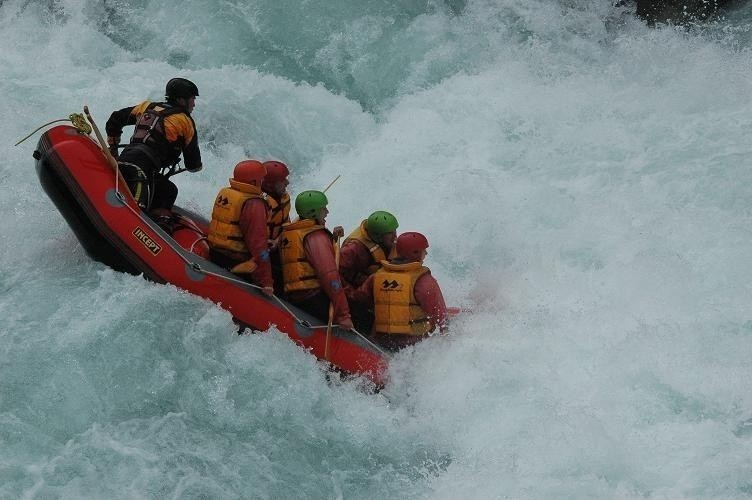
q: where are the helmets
[259,160,290,198]
[295,190,328,222]
[396,231,429,262]
[365,211,398,244]
[165,78,199,108]
[233,160,267,190]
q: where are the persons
[262,161,291,244]
[339,232,449,350]
[207,160,273,297]
[106,77,202,210]
[339,211,399,288]
[281,190,354,332]
